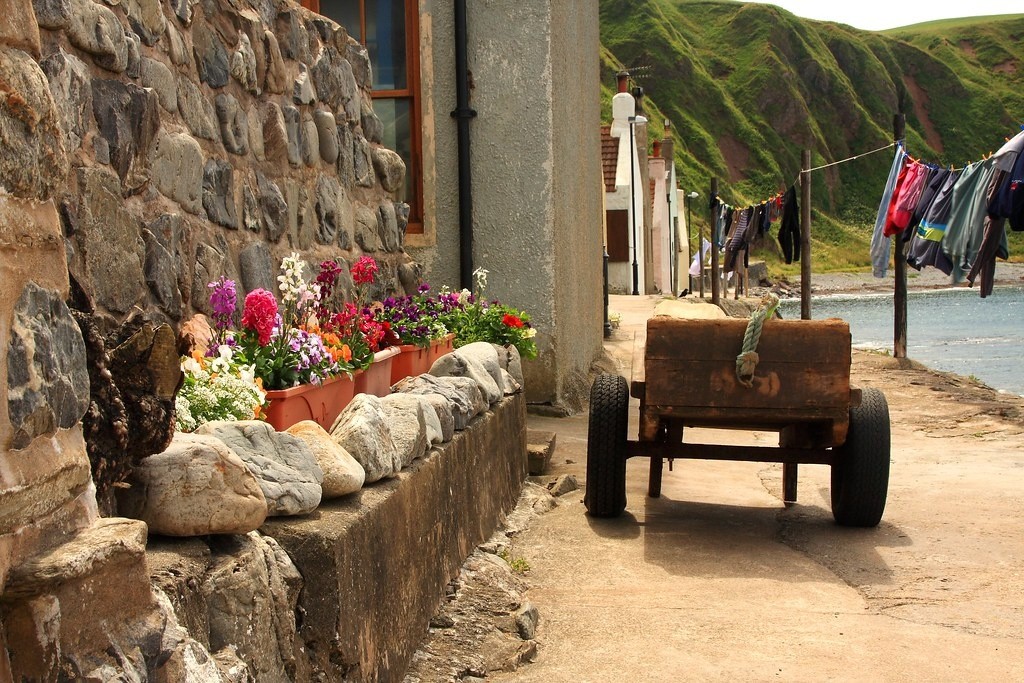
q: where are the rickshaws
[583,314,891,526]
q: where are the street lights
[688,192,699,294]
[628,116,647,296]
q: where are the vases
[391,334,456,385]
[353,346,401,398]
[262,368,364,432]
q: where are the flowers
[173,253,538,433]
